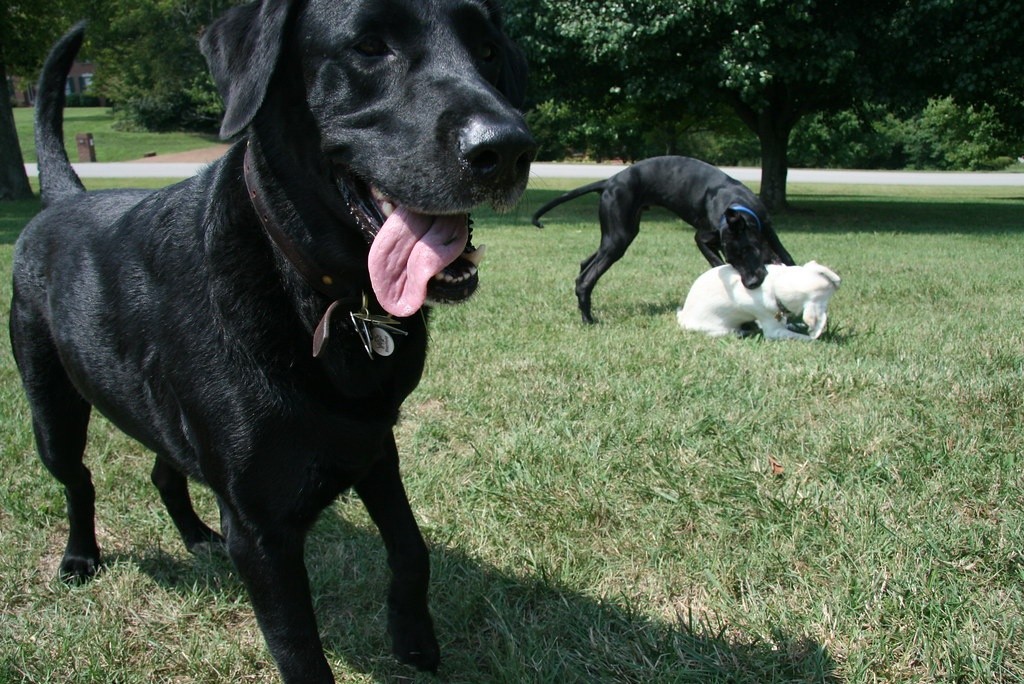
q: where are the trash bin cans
[75,132,96,162]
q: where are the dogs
[532,156,851,350]
[0,0,552,684]
